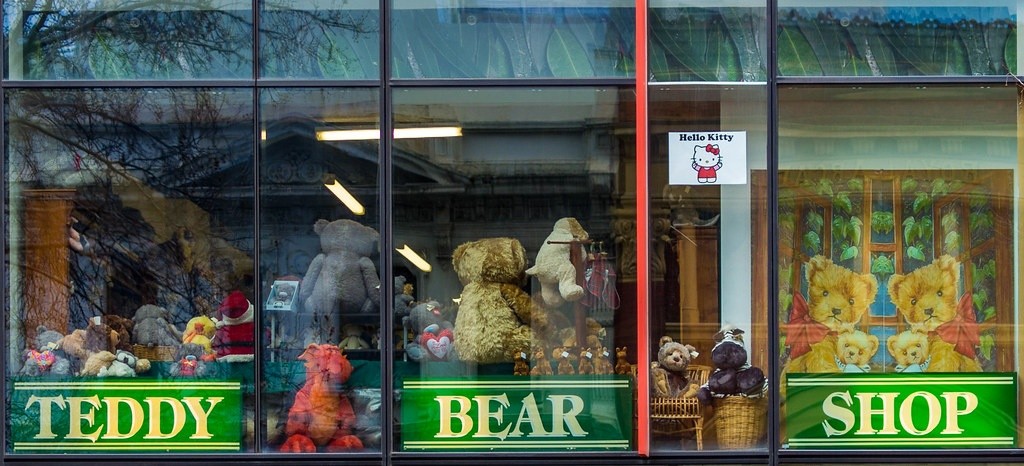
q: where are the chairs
[631,360,712,452]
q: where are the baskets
[133,345,179,361]
[712,396,768,450]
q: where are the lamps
[324,178,365,215]
[395,241,433,273]
[313,120,462,141]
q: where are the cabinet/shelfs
[21,189,109,347]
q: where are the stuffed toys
[395,217,632,375]
[652,326,765,400]
[22,289,254,377]
[282,343,363,453]
[262,219,380,349]
